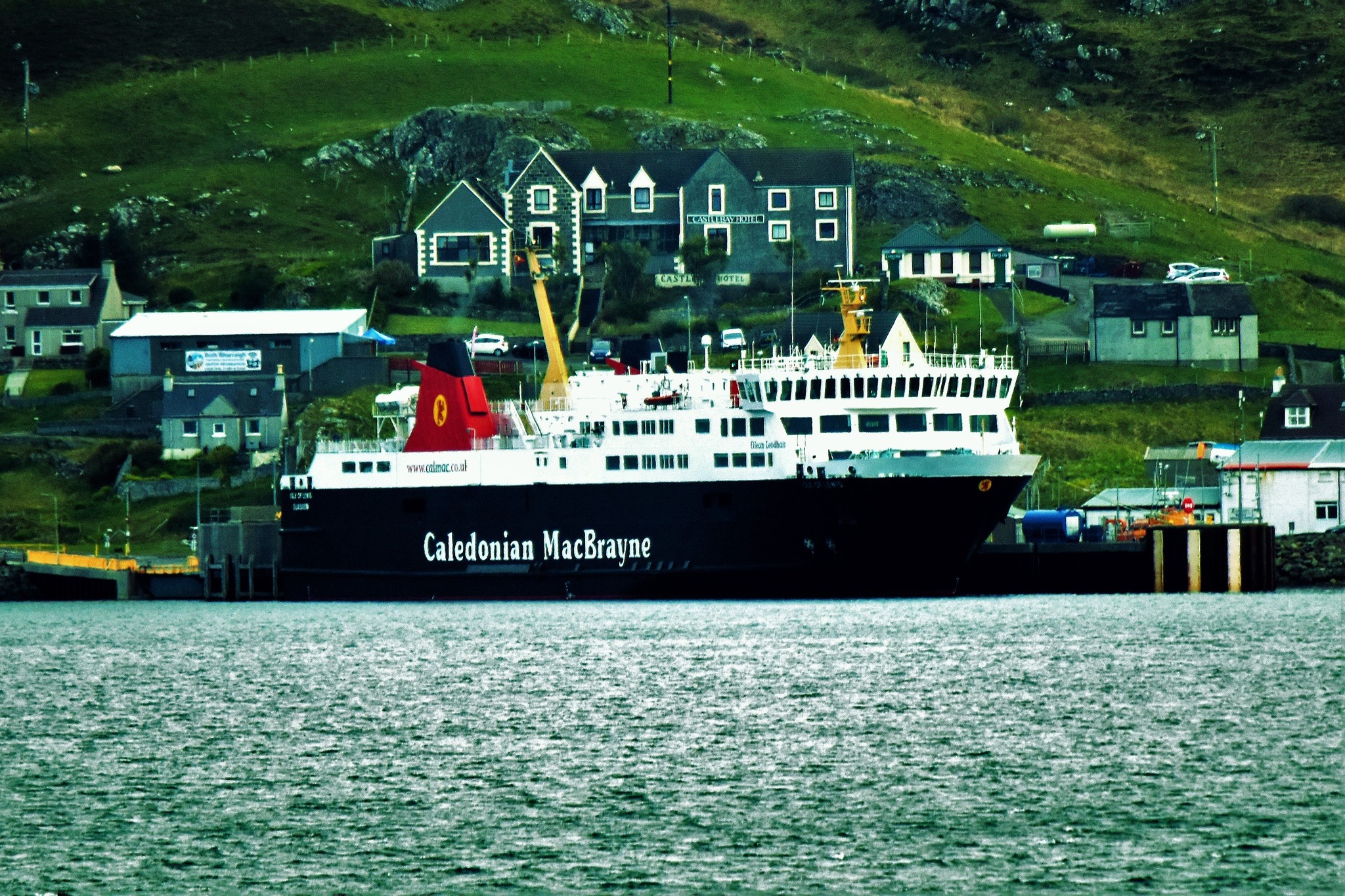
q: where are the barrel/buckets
[1021,506,1083,543]
[1089,525,1103,542]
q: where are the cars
[759,329,777,343]
[590,340,614,364]
[511,339,549,361]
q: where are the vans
[719,328,746,354]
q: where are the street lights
[1200,124,1224,219]
[684,296,691,361]
[39,493,59,565]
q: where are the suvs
[1174,267,1230,283]
[1165,263,1199,280]
[464,333,510,357]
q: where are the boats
[644,393,680,406]
[269,236,1045,602]
